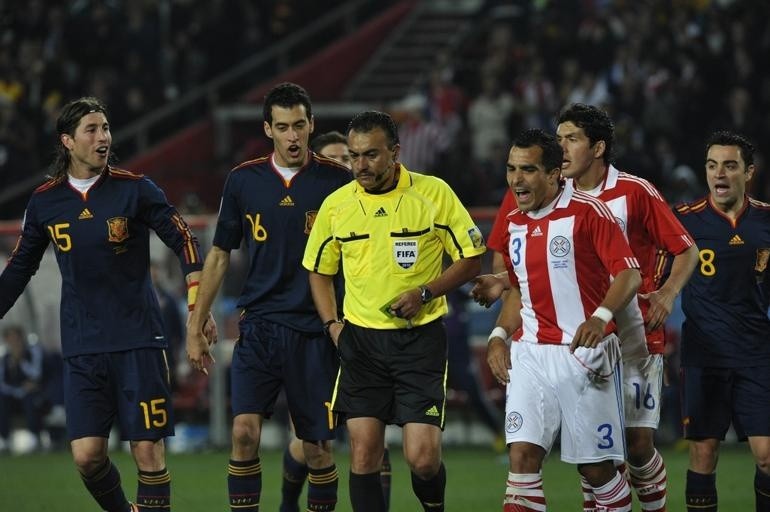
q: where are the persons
[0,324,50,455]
[279,131,391,511]
[188,82,353,512]
[468,106,700,511]
[657,133,770,512]
[0,98,218,511]
[0,1,149,187]
[302,111,485,511]
[146,259,188,397]
[485,131,644,512]
[396,0,770,209]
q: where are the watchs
[418,284,432,304]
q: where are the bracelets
[321,316,343,335]
